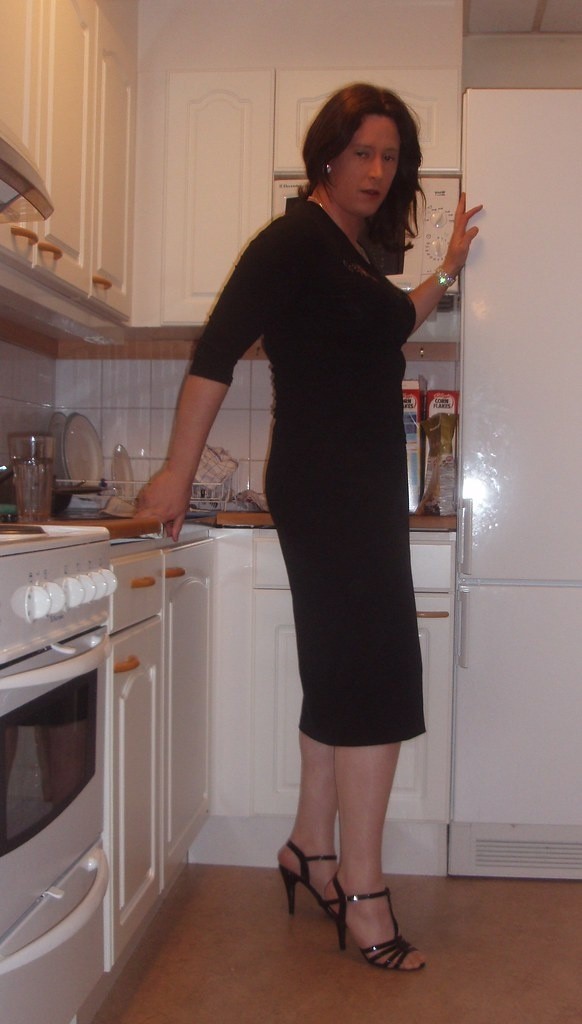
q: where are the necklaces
[308,196,324,210]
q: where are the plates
[111,444,134,495]
[47,409,66,476]
[63,413,104,483]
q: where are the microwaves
[276,178,460,294]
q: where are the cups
[9,435,52,521]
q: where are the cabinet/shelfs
[130,0,464,340]
[1,0,136,364]
[96,526,218,970]
[239,526,453,826]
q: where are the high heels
[324,875,425,972]
[277,840,343,922]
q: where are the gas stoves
[0,520,116,675]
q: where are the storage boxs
[404,374,462,516]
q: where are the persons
[133,83,483,971]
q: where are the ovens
[0,621,124,1024]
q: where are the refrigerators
[448,90,582,882]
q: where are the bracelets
[436,268,455,286]
